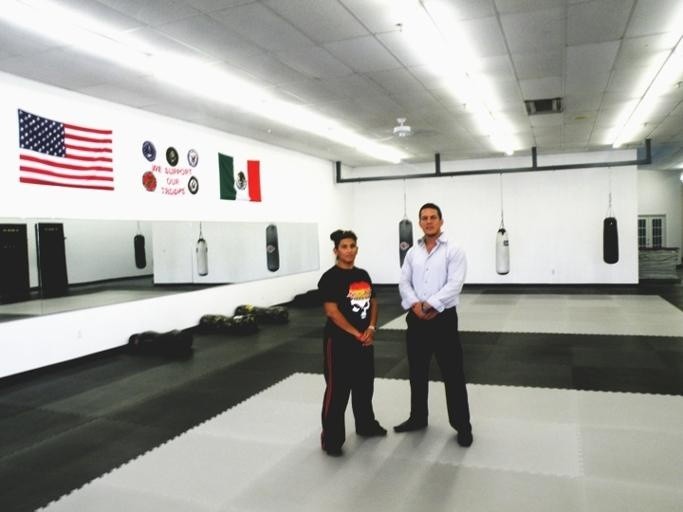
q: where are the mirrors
[0,216,321,325]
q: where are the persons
[317,230,387,457]
[392,203,473,447]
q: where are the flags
[17,109,114,190]
[217,151,261,202]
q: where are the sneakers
[326,446,343,455]
[367,425,386,436]
[394,418,428,432]
[457,425,472,446]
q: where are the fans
[368,118,443,144]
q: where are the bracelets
[355,332,362,342]
[367,325,377,332]
